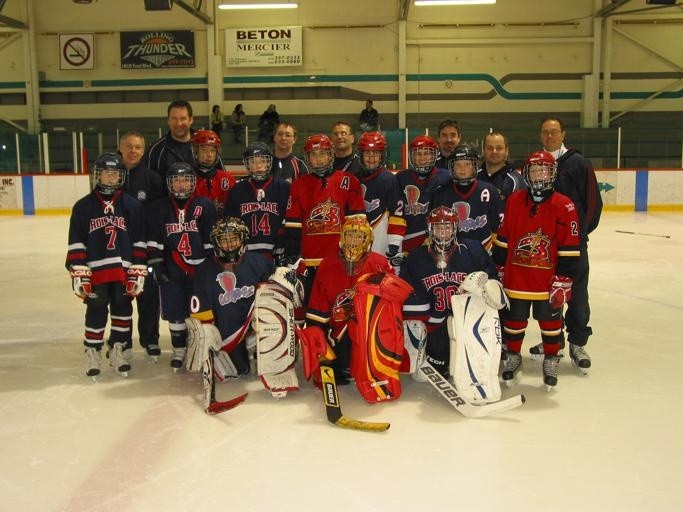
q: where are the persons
[145,101,227,180]
[210,103,280,144]
[224,141,292,260]
[189,129,238,219]
[498,116,602,384]
[105,131,165,356]
[146,161,218,367]
[357,97,380,126]
[352,115,529,274]
[187,216,278,379]
[265,120,363,180]
[396,206,501,382]
[303,216,398,389]
[66,150,146,376]
[283,132,368,310]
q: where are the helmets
[91,153,126,194]
[209,217,250,265]
[425,205,459,246]
[340,216,374,262]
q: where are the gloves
[548,274,575,309]
[146,257,170,290]
[69,265,98,303]
[122,264,148,298]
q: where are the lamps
[217,1,299,10]
[414,1,497,7]
